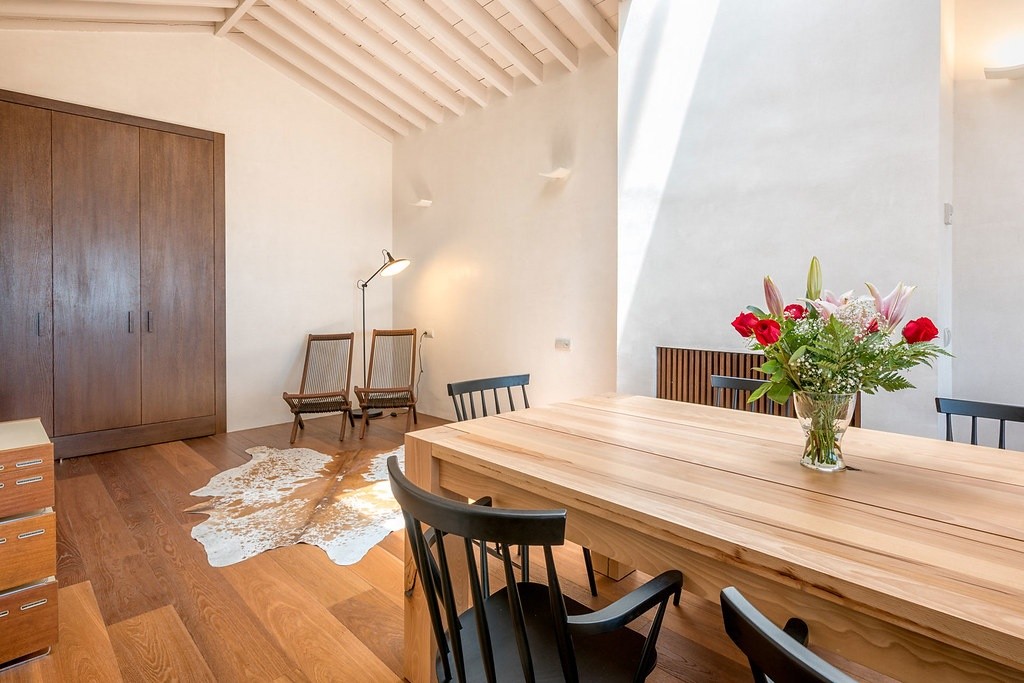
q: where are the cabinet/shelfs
[0,417,60,673]
[0,89,228,460]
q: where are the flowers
[731,256,955,466]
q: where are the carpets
[183,444,406,565]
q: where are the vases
[791,389,858,473]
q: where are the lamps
[350,249,410,418]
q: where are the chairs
[720,586,858,683]
[935,397,1024,451]
[447,376,598,602]
[387,455,684,683]
[282,333,355,443]
[353,328,417,440]
[672,375,790,607]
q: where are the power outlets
[424,330,433,338]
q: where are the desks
[402,392,1024,683]
